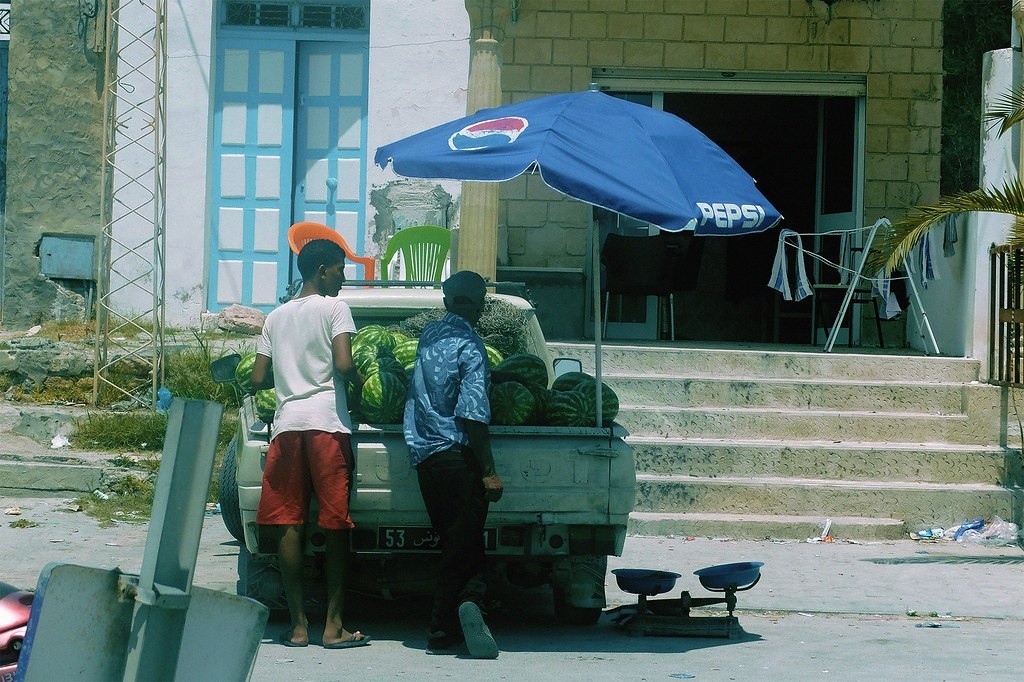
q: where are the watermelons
[234,324,621,425]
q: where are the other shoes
[458,602,500,660]
[426,630,470,655]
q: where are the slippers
[280,631,309,647]
[323,631,375,648]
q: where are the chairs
[380,225,451,288]
[288,222,375,289]
[810,248,884,350]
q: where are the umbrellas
[374,83,785,428]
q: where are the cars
[0,581,36,682]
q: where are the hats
[443,270,487,308]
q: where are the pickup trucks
[208,277,638,629]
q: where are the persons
[403,270,504,659]
[252,239,368,649]
